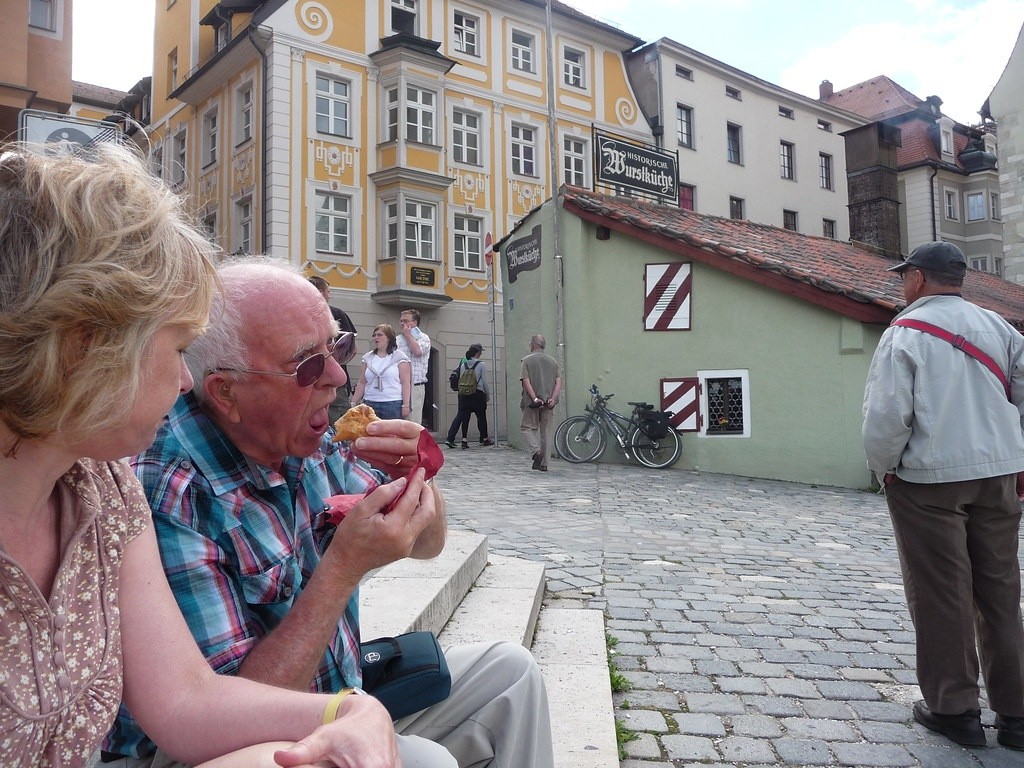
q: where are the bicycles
[555,384,683,468]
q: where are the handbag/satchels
[449,357,464,391]
[361,631,451,721]
[646,411,669,440]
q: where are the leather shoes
[993,713,1024,750]
[913,699,986,746]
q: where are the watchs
[322,687,368,724]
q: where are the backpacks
[458,361,482,396]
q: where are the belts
[414,383,424,386]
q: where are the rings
[394,455,403,466]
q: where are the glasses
[899,268,927,283]
[372,332,385,337]
[208,331,356,388]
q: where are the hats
[475,343,485,351]
[887,240,967,277]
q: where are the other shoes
[532,451,543,470]
[445,440,458,448]
[479,439,483,445]
[539,466,547,471]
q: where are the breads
[331,403,382,442]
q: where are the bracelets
[533,398,538,402]
[401,405,410,408]
[350,401,357,405]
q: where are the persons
[461,345,494,449]
[519,335,562,471]
[0,119,462,768]
[309,275,358,429]
[394,309,431,426]
[348,324,412,420]
[445,343,486,448]
[861,240,1024,749]
[100,253,557,768]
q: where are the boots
[483,439,495,446]
[461,441,470,449]
